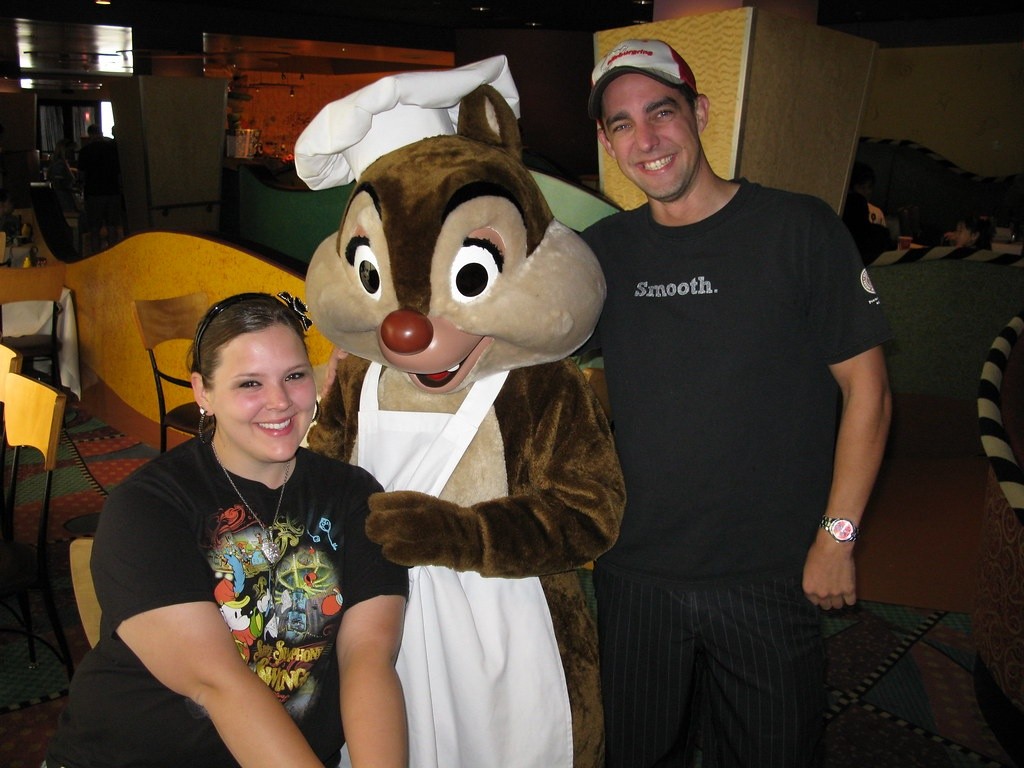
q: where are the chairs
[0,230,76,684]
[131,290,214,453]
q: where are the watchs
[818,515,861,544]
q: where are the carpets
[0,359,165,716]
[577,561,1023,768]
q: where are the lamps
[280,72,287,80]
[299,74,305,80]
[290,87,296,99]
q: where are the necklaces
[211,439,290,562]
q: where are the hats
[588,39,698,127]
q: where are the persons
[0,188,21,244]
[47,139,82,256]
[76,122,121,252]
[325,39,893,768]
[38,293,411,768]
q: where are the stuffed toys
[294,54,630,768]
